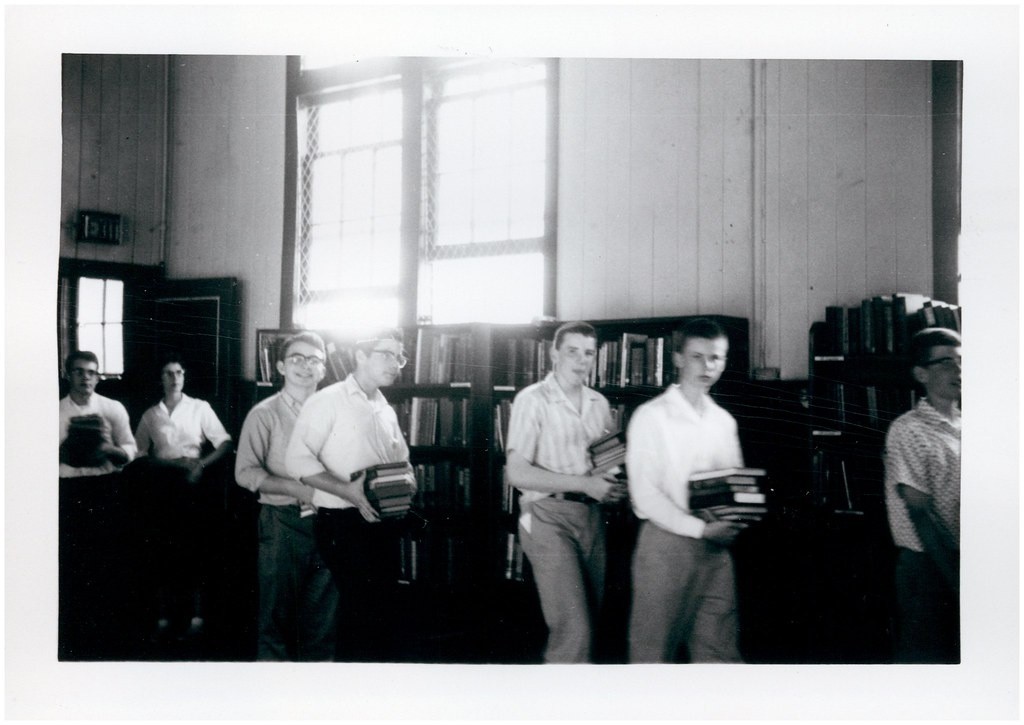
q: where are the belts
[548,491,599,507]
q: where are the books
[823,294,959,428]
[256,317,768,585]
[67,414,116,449]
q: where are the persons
[625,319,747,663]
[133,361,232,634]
[237,331,326,662]
[286,325,418,663]
[505,321,629,664]
[883,326,960,664]
[59,351,143,479]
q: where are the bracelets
[199,459,205,468]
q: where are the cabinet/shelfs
[253,313,750,583]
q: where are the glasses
[70,368,101,380]
[282,352,326,369]
[369,348,407,368]
[923,356,961,369]
[162,369,185,380]
